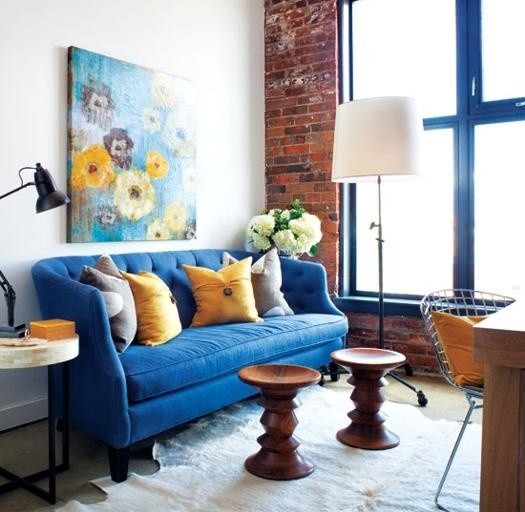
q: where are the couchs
[28,247,348,483]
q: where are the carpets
[52,384,482,512]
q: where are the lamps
[0,163,74,339]
[330,94,430,407]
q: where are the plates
[0,338,47,347]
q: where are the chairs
[417,286,518,512]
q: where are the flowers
[241,200,324,261]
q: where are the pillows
[221,246,295,317]
[120,268,184,347]
[179,256,264,330]
[434,306,495,390]
[75,251,139,356]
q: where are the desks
[1,332,83,507]
[472,291,525,511]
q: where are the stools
[332,345,409,452]
[234,361,321,482]
